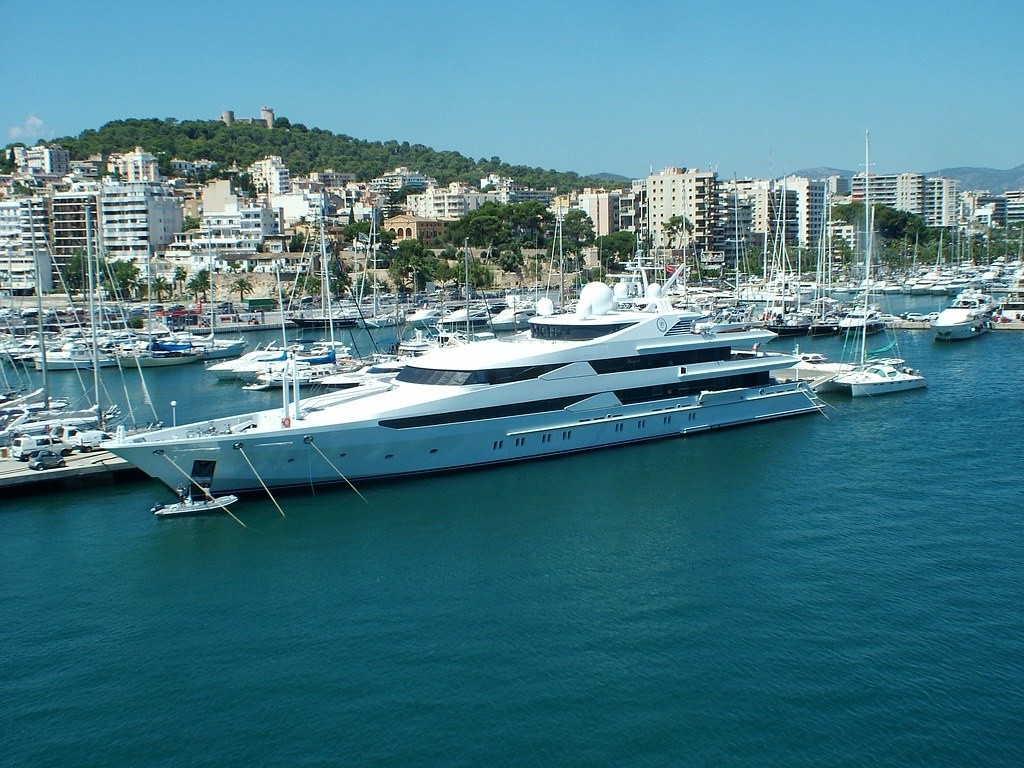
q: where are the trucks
[242,297,278,313]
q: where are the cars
[68,308,89,317]
[21,307,44,317]
[47,309,67,316]
[300,296,320,310]
[144,304,164,313]
[429,293,445,302]
[330,294,346,304]
[282,298,294,310]
[217,301,233,308]
[361,293,375,304]
[413,291,428,302]
[95,307,111,315]
[126,306,146,315]
[169,304,185,312]
[484,294,495,299]
[378,292,397,306]
[398,291,411,304]
[188,303,205,313]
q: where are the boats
[0,128,1024,520]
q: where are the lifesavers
[283,418,291,427]
